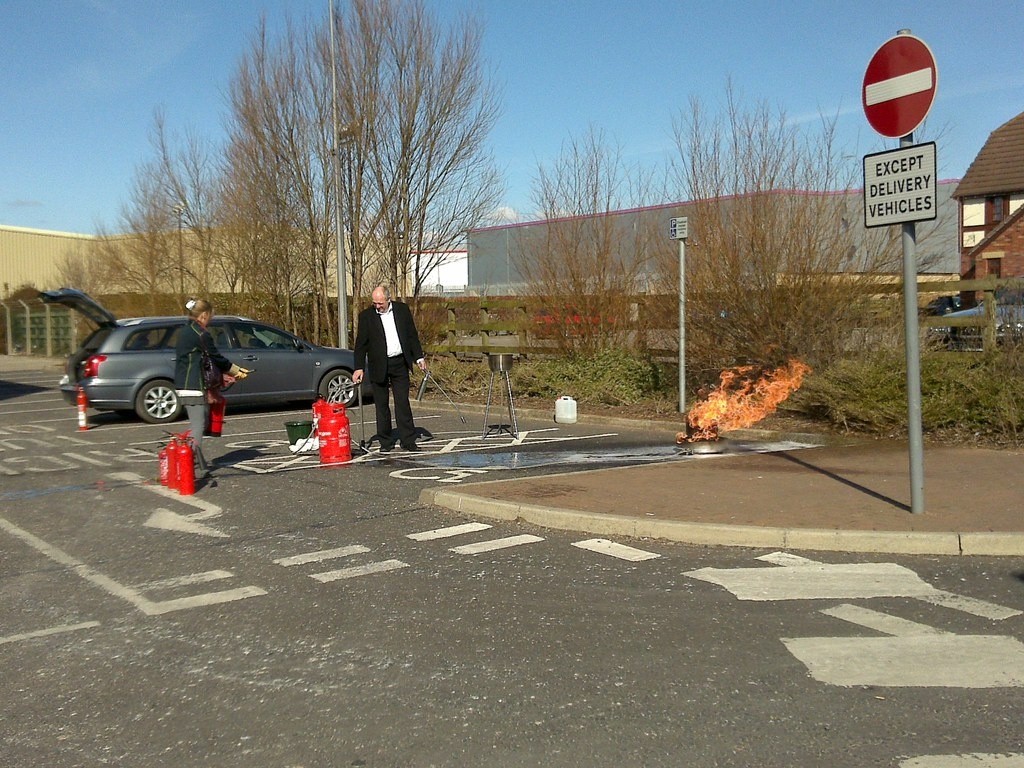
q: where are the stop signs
[858,33,940,141]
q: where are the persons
[352,286,427,451]
[174,300,249,479]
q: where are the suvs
[37,287,375,423]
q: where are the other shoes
[401,444,421,451]
[380,444,394,452]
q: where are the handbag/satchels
[199,331,223,390]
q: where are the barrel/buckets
[554,395,578,424]
[283,420,315,446]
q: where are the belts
[387,354,404,359]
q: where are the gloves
[226,363,249,380]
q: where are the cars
[923,285,1023,352]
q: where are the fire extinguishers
[156,428,196,496]
[76,385,89,431]
[203,368,257,437]
[415,369,467,425]
[313,392,353,464]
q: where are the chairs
[216,332,228,350]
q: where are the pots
[481,351,514,372]
